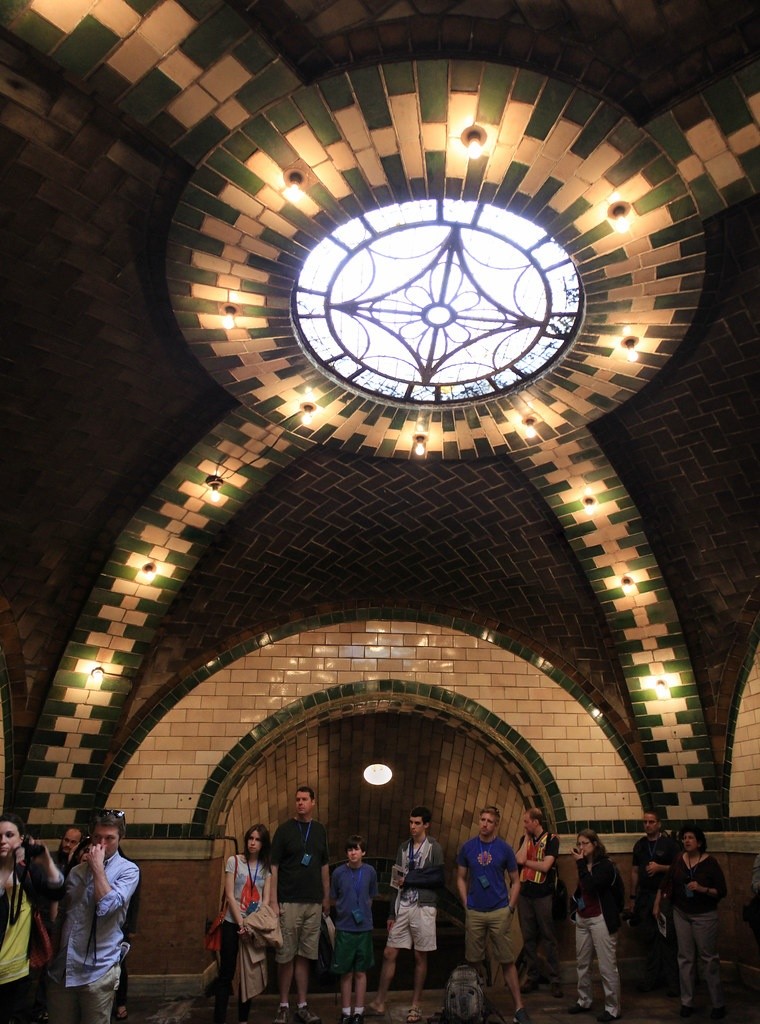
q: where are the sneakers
[513,1008,531,1024]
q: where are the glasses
[97,809,125,826]
[81,834,90,842]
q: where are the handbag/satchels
[205,911,226,952]
[17,863,52,968]
[548,833,568,921]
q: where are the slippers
[117,1006,127,1020]
[353,1003,385,1016]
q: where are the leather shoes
[569,1003,591,1014]
[680,1004,694,1017]
[667,983,680,997]
[340,1013,352,1024]
[637,979,661,991]
[352,1011,365,1024]
[520,978,539,994]
[597,1011,620,1021]
[711,1005,726,1018]
[551,982,564,998]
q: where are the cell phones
[573,848,578,854]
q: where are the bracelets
[509,906,515,913]
[706,888,710,893]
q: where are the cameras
[21,835,45,865]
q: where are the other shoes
[294,1005,322,1024]
[274,1005,290,1024]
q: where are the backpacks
[442,964,490,1024]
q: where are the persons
[456,806,532,1024]
[630,810,680,960]
[567,828,624,1023]
[652,827,730,1024]
[751,854,760,894]
[269,787,330,1024]
[514,808,565,999]
[363,806,446,1023]
[212,824,272,1024]
[331,835,379,1024]
[0,808,143,1024]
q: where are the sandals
[406,1007,423,1023]
